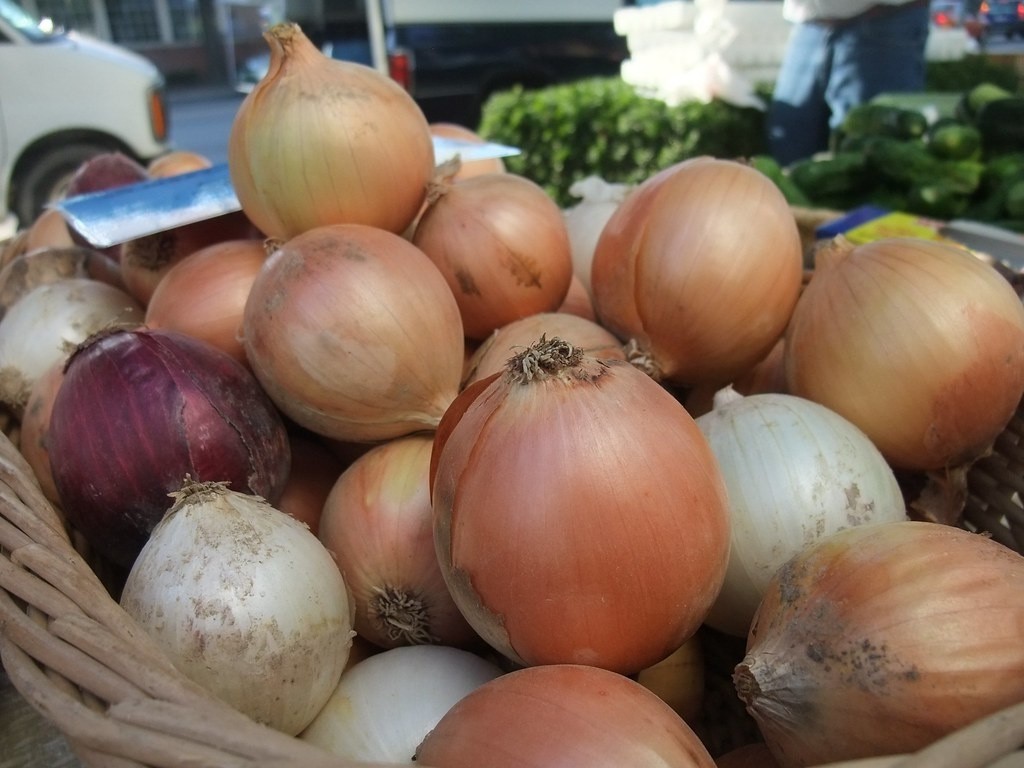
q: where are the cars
[1,0,170,225]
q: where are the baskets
[1,182,1024,768]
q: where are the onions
[0,20,1024,768]
